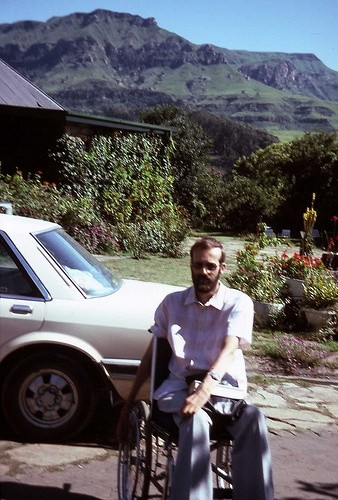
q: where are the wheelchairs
[116,335,234,500]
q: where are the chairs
[277,230,290,238]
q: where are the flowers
[282,252,324,279]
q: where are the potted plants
[251,276,284,327]
[299,277,338,327]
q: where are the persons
[115,235,276,500]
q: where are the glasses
[191,261,221,271]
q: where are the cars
[0,212,189,443]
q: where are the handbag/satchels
[188,379,248,427]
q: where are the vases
[284,277,304,299]
